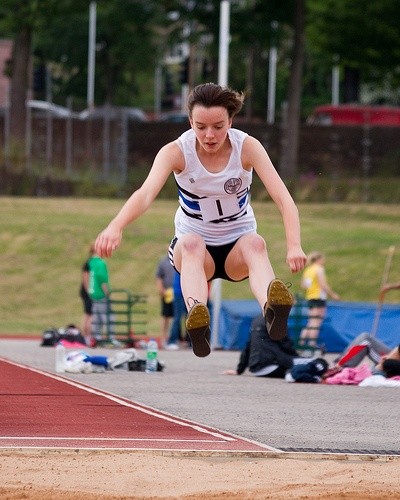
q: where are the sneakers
[186,302,212,358]
[264,278,295,340]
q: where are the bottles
[54,341,68,373]
[144,337,159,374]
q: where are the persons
[94,84,307,357]
[221,315,400,380]
[301,251,339,348]
[80,249,188,349]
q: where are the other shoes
[110,337,121,347]
[160,337,188,351]
[85,337,97,347]
